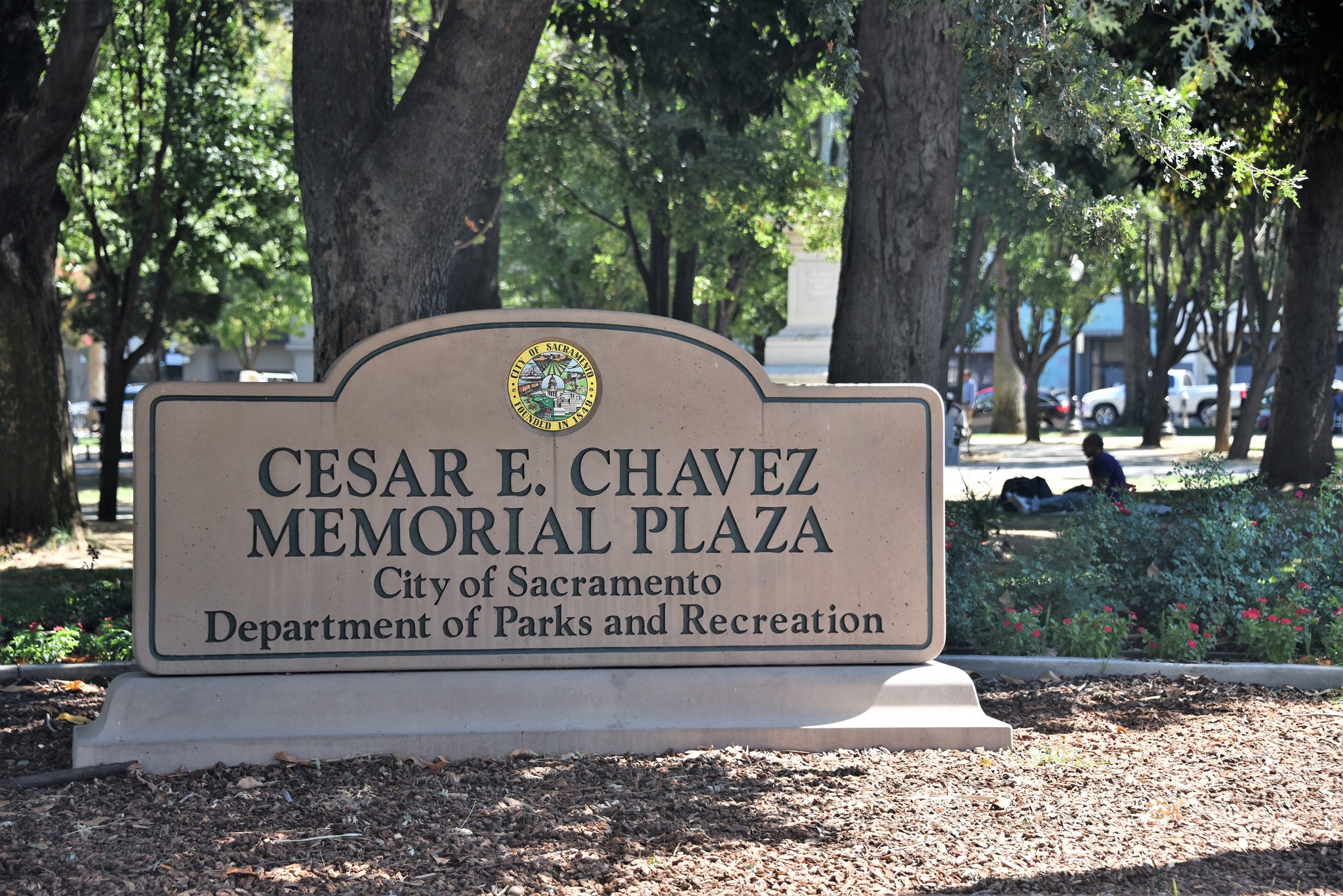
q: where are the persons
[1005,433,1126,516]
[960,369,976,439]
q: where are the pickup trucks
[1081,369,1246,429]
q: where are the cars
[946,384,1076,434]
[90,381,144,458]
[1204,379,1343,433]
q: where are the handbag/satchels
[1003,476,1053,501]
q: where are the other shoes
[1010,493,1030,515]
